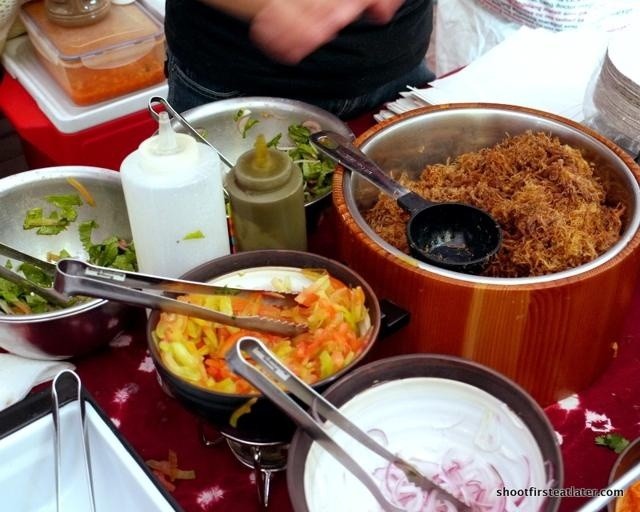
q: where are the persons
[163,0,436,124]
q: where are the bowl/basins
[144,253,381,427]
[341,106,640,286]
[154,97,358,212]
[287,352,564,511]
[0,164,127,362]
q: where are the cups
[43,0,112,27]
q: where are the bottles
[117,113,229,304]
[229,132,308,251]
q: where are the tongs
[46,257,311,342]
[0,241,69,307]
[48,370,97,511]
[227,334,471,512]
